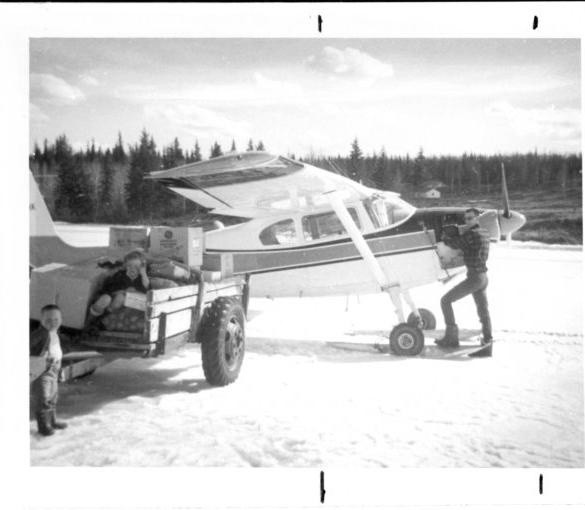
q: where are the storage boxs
[150,227,203,267]
[109,228,147,263]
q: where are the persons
[430,207,494,359]
[31,303,68,437]
[68,249,151,352]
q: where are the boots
[468,338,492,356]
[85,312,98,332]
[435,325,459,347]
[34,409,67,436]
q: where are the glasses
[462,216,476,220]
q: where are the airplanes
[30,150,527,358]
